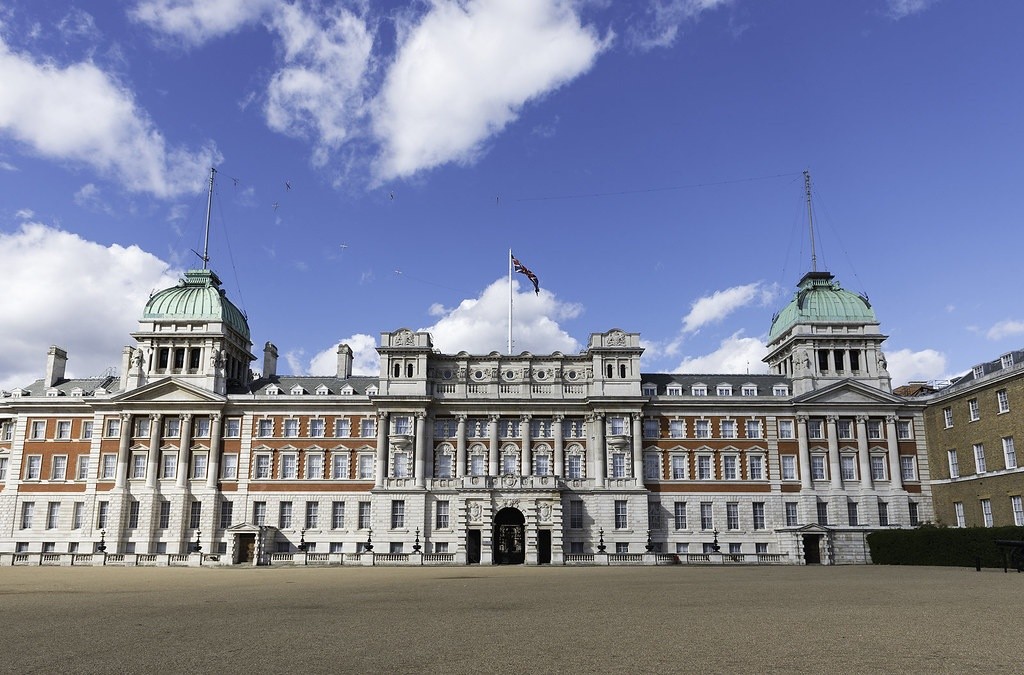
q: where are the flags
[511,256,540,296]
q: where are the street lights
[298,531,306,551]
[597,527,606,552]
[194,531,202,552]
[412,527,421,552]
[712,527,720,552]
[645,531,654,552]
[98,530,106,552]
[364,530,373,551]
